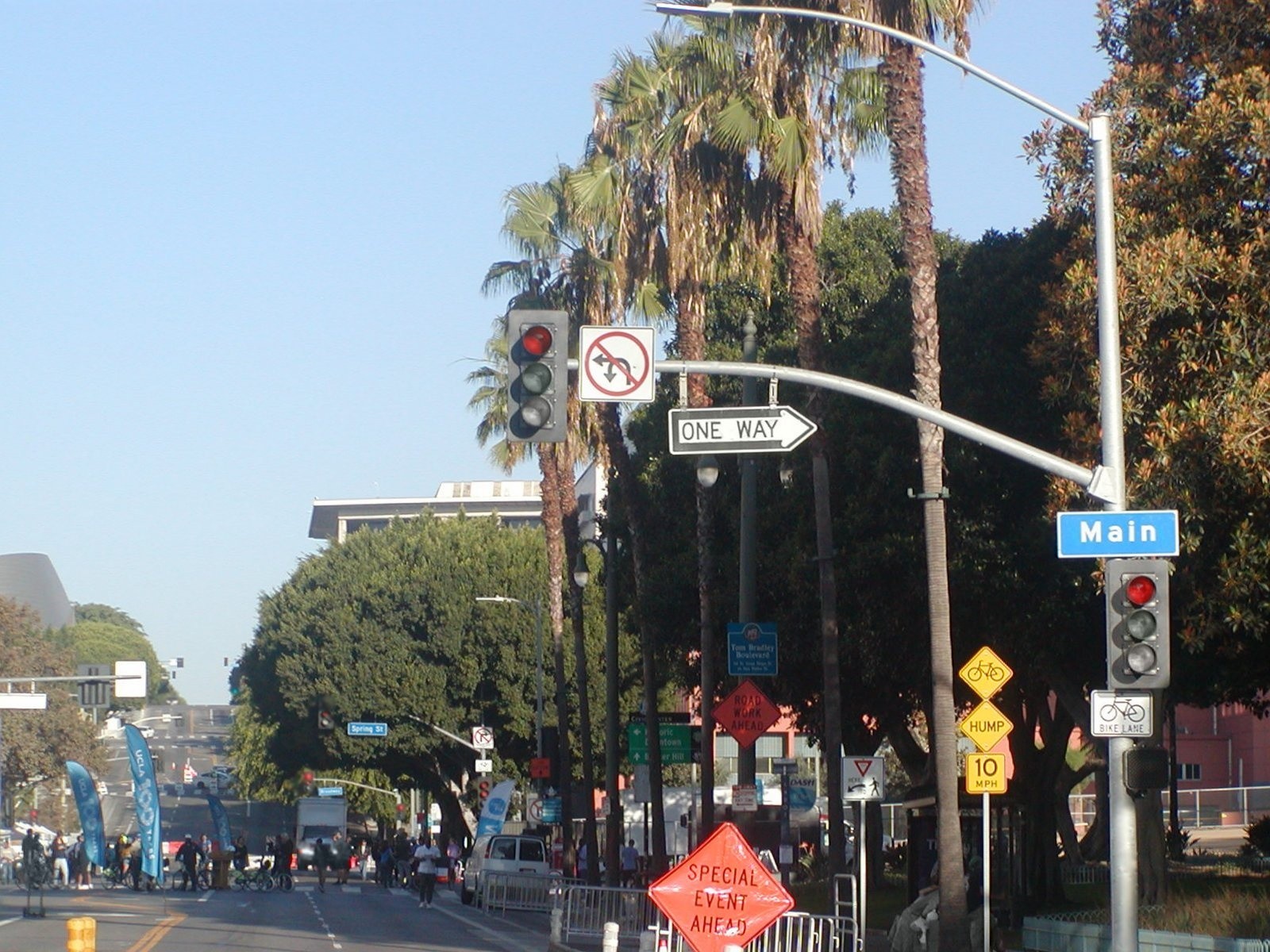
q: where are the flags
[65,760,107,867]
[206,794,232,850]
[125,722,164,886]
[476,780,516,835]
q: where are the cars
[193,763,241,790]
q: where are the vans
[461,835,548,905]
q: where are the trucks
[296,797,349,871]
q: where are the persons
[331,828,462,891]
[576,838,588,899]
[621,839,639,888]
[887,837,981,952]
[415,835,441,909]
[312,837,331,893]
[0,828,294,891]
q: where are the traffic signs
[629,724,692,749]
[841,755,884,804]
[667,406,818,456]
[629,713,692,723]
[629,746,693,764]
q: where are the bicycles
[228,850,295,892]
[12,852,68,891]
[101,855,213,892]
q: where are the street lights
[654,0,1142,952]
[474,595,545,836]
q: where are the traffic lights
[231,684,239,695]
[475,774,495,811]
[398,803,407,820]
[508,307,569,444]
[1105,559,1171,691]
[317,706,333,728]
[303,768,315,793]
[31,808,38,826]
[1123,743,1172,793]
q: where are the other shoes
[426,903,431,909]
[333,880,348,886]
[54,884,93,890]
[419,902,424,908]
